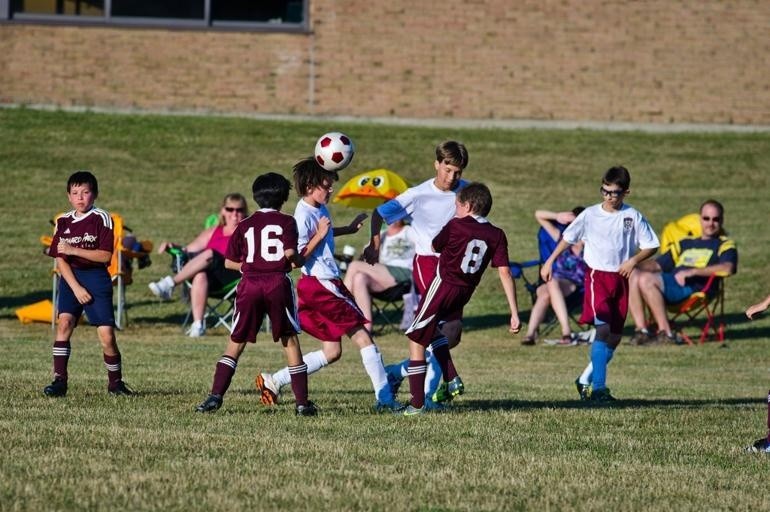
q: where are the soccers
[315,132,355,171]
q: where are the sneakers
[520,334,534,344]
[188,320,203,338]
[108,381,132,399]
[255,373,279,405]
[295,402,317,417]
[591,388,616,401]
[630,331,684,345]
[148,275,176,297]
[557,333,577,346]
[197,394,223,414]
[432,376,463,402]
[572,377,592,401]
[745,436,770,453]
[44,381,67,396]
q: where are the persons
[44,171,134,398]
[522,206,587,347]
[539,167,662,406]
[628,200,737,344]
[254,155,404,413]
[194,174,317,418]
[389,182,522,417]
[342,219,414,335]
[149,193,247,339]
[363,140,471,415]
[745,294,770,454]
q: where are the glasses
[701,215,721,222]
[223,206,246,212]
[599,186,625,197]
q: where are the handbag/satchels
[399,272,424,330]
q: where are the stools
[368,277,414,335]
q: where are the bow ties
[377,361,445,415]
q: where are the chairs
[649,212,735,342]
[39,210,153,331]
[509,223,591,340]
[166,212,252,335]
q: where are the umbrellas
[334,171,410,208]
